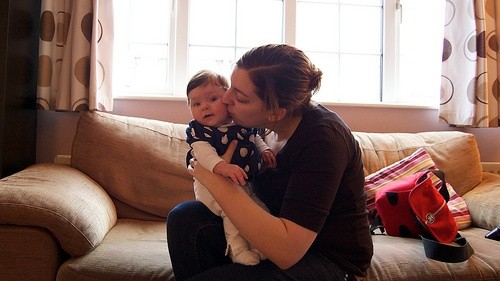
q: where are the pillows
[364,147,472,234]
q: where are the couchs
[0,112,500,281]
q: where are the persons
[167,43,374,281]
[185,70,278,266]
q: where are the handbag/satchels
[372,170,459,245]
